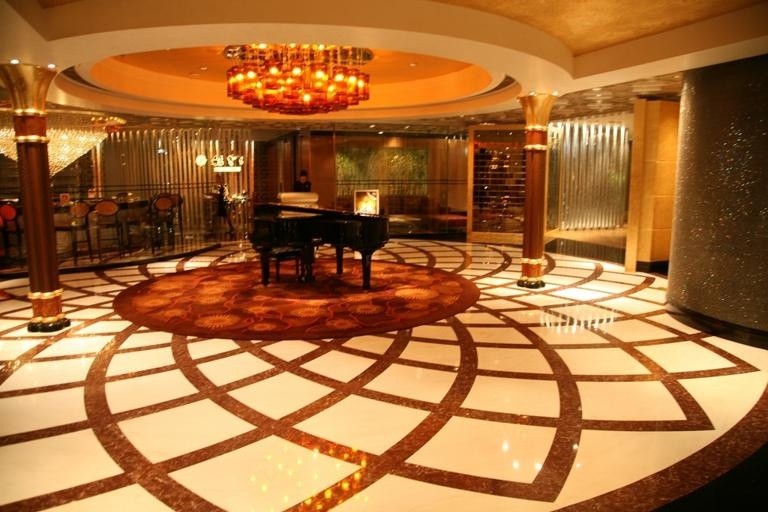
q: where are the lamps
[222,44,374,115]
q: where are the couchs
[337,195,467,238]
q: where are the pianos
[248,200,389,291]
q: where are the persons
[294,170,312,192]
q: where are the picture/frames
[354,189,379,215]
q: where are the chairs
[0,192,184,269]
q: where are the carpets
[111,256,481,341]
